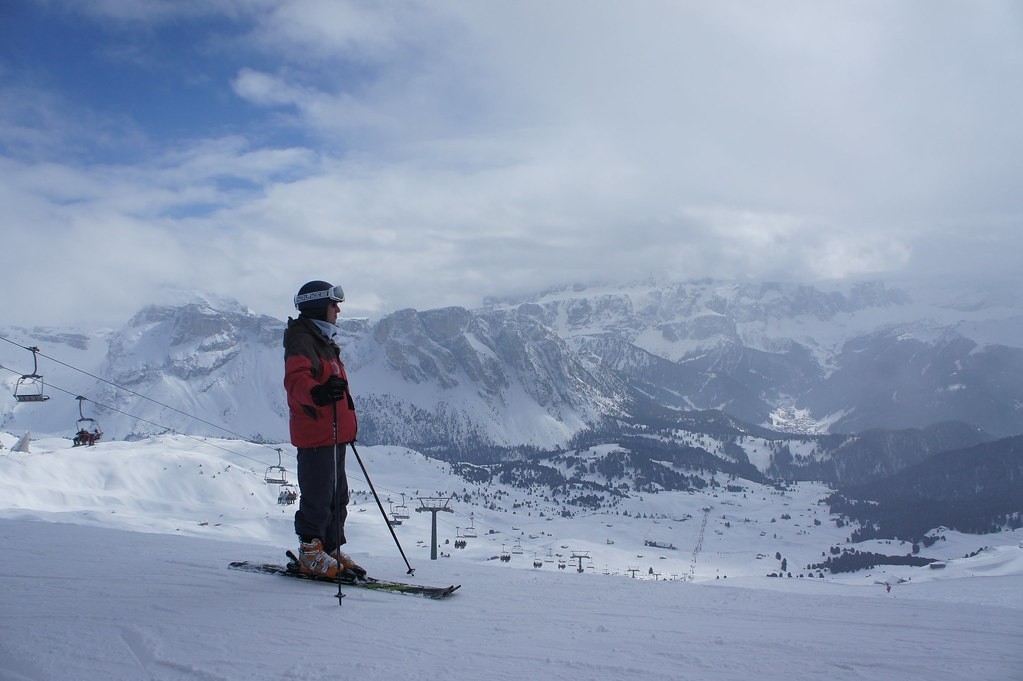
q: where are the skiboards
[227,561,461,598]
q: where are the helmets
[298,281,334,321]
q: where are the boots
[298,539,367,584]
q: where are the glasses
[295,285,345,305]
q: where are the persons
[278,490,297,506]
[454,539,466,549]
[558,563,566,569]
[73,428,101,445]
[283,280,366,581]
[500,555,511,562]
[534,561,542,568]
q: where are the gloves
[312,375,348,406]
